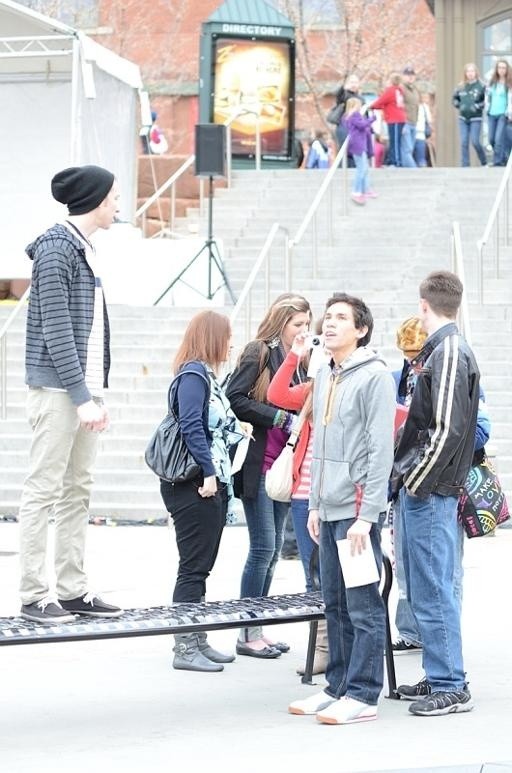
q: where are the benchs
[0,553,401,700]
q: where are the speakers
[194,122,227,179]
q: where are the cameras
[303,335,325,350]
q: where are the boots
[172,632,224,672]
[297,619,329,675]
[196,632,235,663]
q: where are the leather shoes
[268,641,290,652]
[236,638,281,658]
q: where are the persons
[452,63,481,167]
[289,294,396,724]
[300,66,435,206]
[483,60,511,165]
[160,312,253,671]
[387,271,479,719]
[146,112,168,154]
[19,165,124,624]
[226,293,314,660]
[265,320,336,677]
[387,315,490,658]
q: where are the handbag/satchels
[265,390,314,503]
[145,360,213,483]
[221,340,272,476]
[457,456,510,538]
[327,103,345,125]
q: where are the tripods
[151,181,238,310]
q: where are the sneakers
[350,190,378,203]
[315,695,377,724]
[57,591,125,618]
[397,672,467,701]
[20,596,76,623]
[384,637,423,656]
[409,681,474,716]
[288,689,339,715]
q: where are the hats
[403,68,415,75]
[396,317,429,351]
[51,165,114,216]
